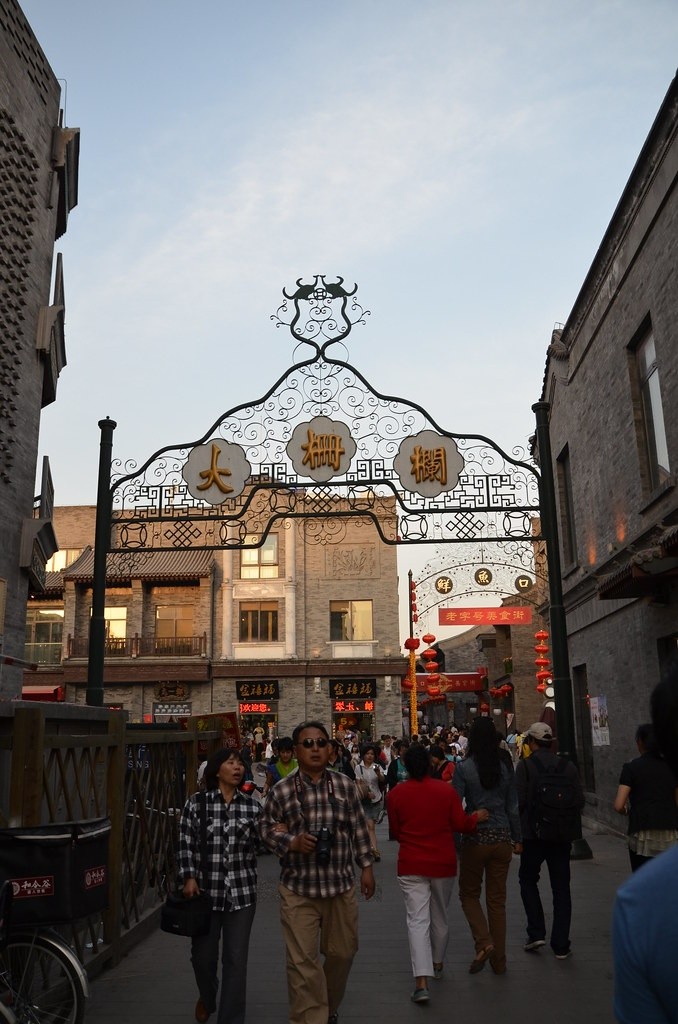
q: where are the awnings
[22,685,61,694]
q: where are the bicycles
[0,816,112,1024]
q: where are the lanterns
[422,634,436,643]
[489,684,512,698]
[422,648,437,658]
[402,678,414,687]
[425,661,438,672]
[412,582,418,622]
[534,629,551,692]
[427,674,440,683]
[418,686,446,708]
[405,638,420,648]
[481,704,489,711]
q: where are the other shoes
[432,966,444,978]
[328,1012,338,1024]
[489,954,506,975]
[371,847,381,862]
[469,943,494,974]
[411,988,430,1002]
[195,982,219,1023]
[375,811,386,824]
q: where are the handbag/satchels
[353,764,369,799]
[159,888,214,937]
[432,760,452,781]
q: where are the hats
[524,721,552,741]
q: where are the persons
[384,744,490,1002]
[516,721,579,960]
[241,693,533,857]
[259,721,376,1024]
[452,716,523,975]
[615,723,678,873]
[175,749,289,1024]
[610,668,678,1024]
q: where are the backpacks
[527,752,584,844]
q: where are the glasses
[297,737,329,748]
[473,715,494,722]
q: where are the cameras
[308,827,335,869]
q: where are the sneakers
[523,935,546,950]
[555,948,572,959]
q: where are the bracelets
[625,806,628,816]
